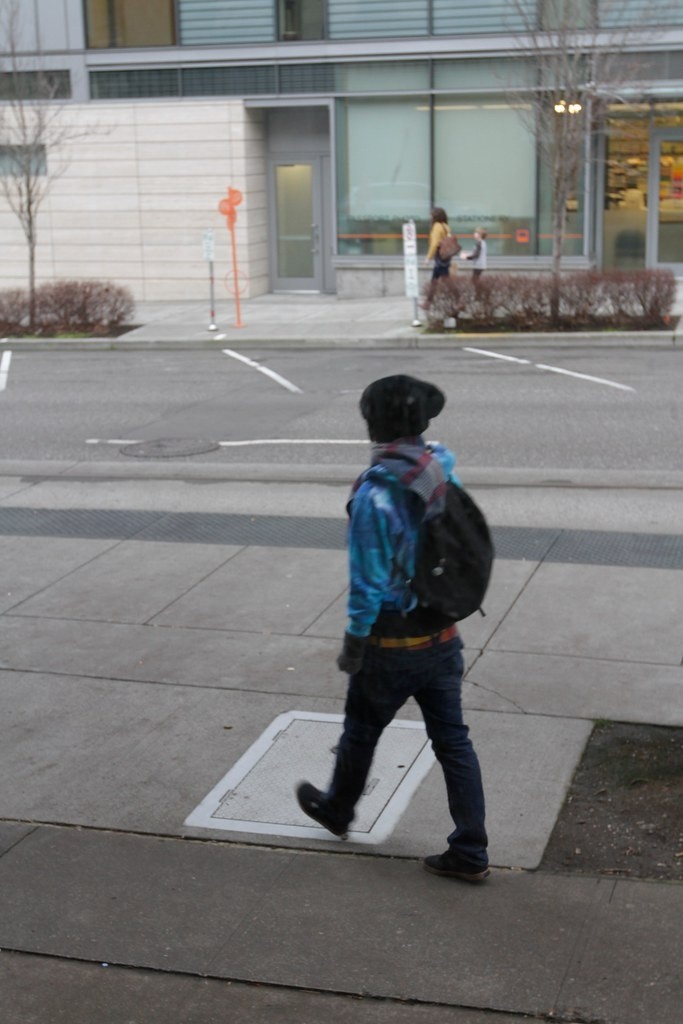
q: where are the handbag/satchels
[436,219,461,260]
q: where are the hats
[360,375,444,441]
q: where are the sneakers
[297,781,348,836]
[423,855,491,883]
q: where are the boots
[418,278,437,310]
[441,277,461,305]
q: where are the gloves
[337,631,369,674]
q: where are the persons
[293,372,491,884]
[459,227,488,301]
[416,207,465,311]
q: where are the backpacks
[396,480,495,638]
